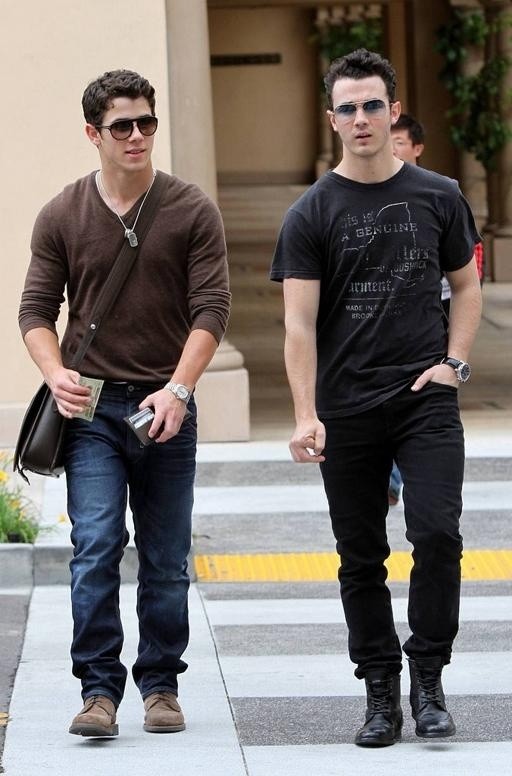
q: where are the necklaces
[96,166,159,251]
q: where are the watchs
[440,356,472,383]
[165,380,192,403]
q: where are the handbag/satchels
[12,380,69,486]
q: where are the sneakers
[69,695,119,737]
[142,692,185,733]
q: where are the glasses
[333,99,395,127]
[96,115,160,141]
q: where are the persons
[19,71,232,738]
[269,50,485,747]
[383,115,484,508]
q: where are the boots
[355,668,403,747]
[406,653,456,739]
